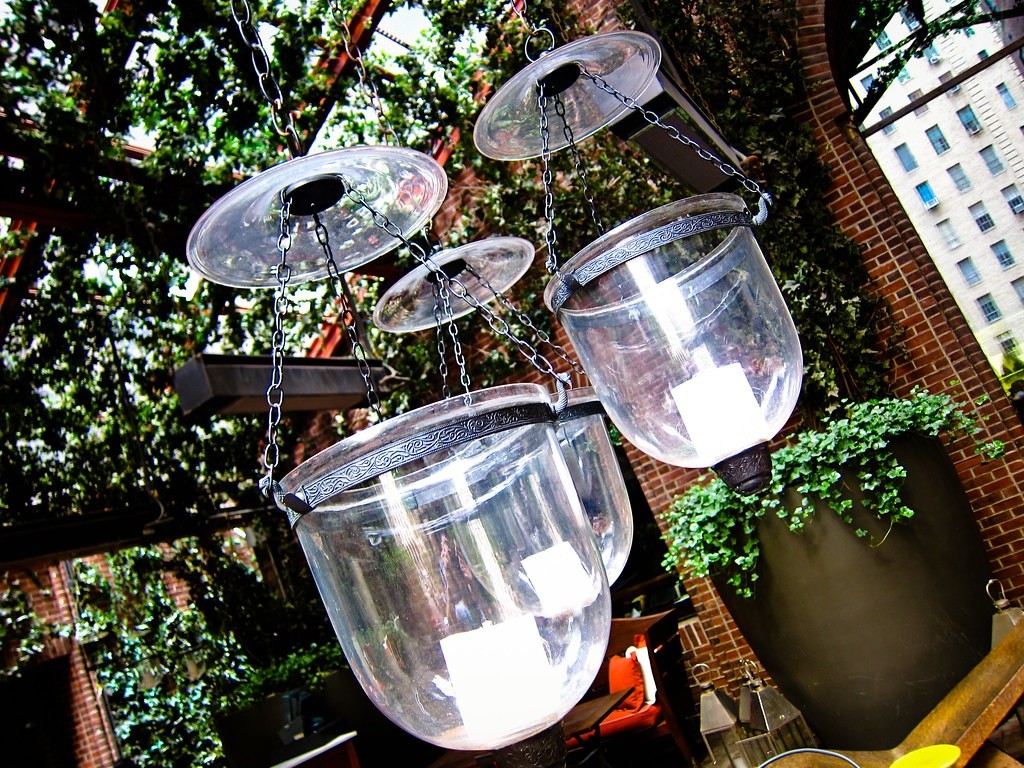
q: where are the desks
[427,687,635,768]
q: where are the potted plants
[661,389,1008,749]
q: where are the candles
[440,613,552,735]
[522,542,594,613]
[672,364,767,457]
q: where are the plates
[888,744,962,768]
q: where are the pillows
[609,646,658,711]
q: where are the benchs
[562,609,697,768]
